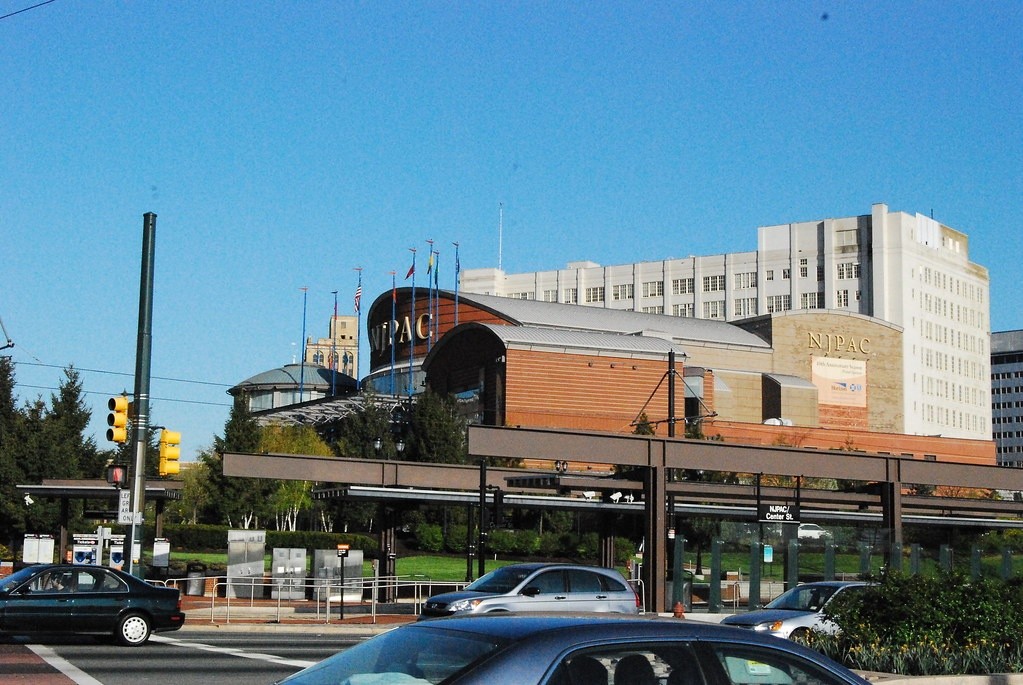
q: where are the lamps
[555,460,568,471]
[373,434,406,461]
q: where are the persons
[29,571,69,593]
[613,654,655,685]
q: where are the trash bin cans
[186,562,207,596]
[664,570,692,613]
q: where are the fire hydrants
[673,603,685,620]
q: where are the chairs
[568,653,660,685]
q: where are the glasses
[51,576,58,580]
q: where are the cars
[272,614,873,685]
[777,523,834,537]
[719,580,882,646]
[0,564,187,645]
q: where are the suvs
[416,562,641,617]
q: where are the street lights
[374,439,405,459]
[555,460,569,476]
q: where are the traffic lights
[107,396,128,443]
[158,428,181,475]
[107,467,129,485]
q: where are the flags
[353,280,361,313]
